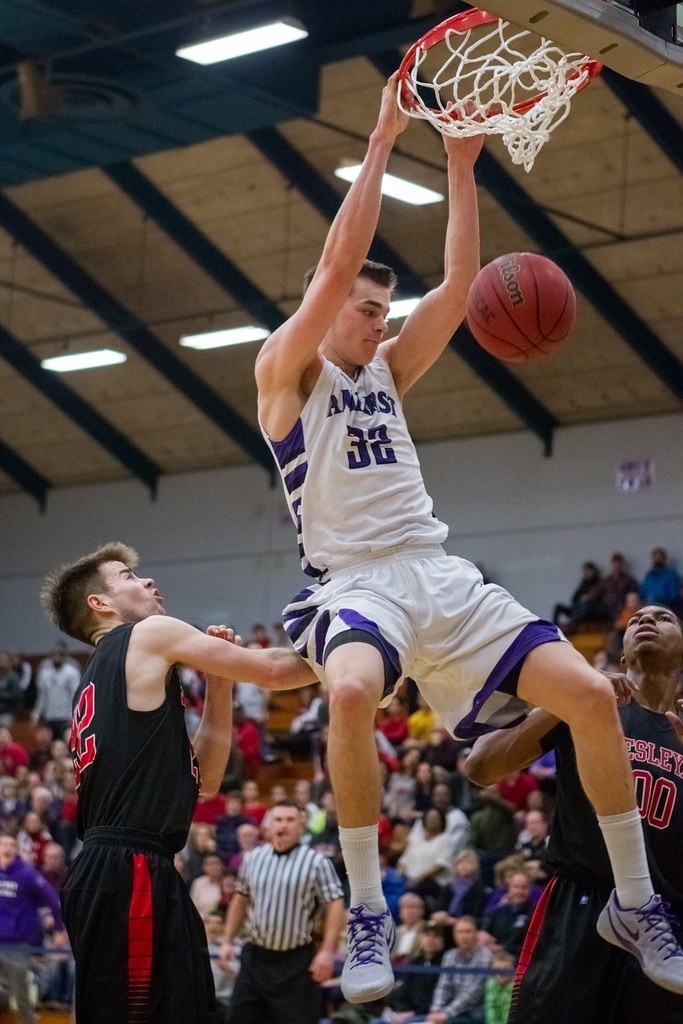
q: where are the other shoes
[36,1001,69,1011]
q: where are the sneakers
[341,903,398,1004]
[597,888,683,995]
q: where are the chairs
[8,687,324,844]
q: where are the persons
[465,604,683,1023]
[40,542,320,1024]
[170,623,561,1024]
[254,71,683,1005]
[472,546,683,718]
[0,639,81,1024]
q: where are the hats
[422,920,443,934]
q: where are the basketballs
[465,250,578,364]
